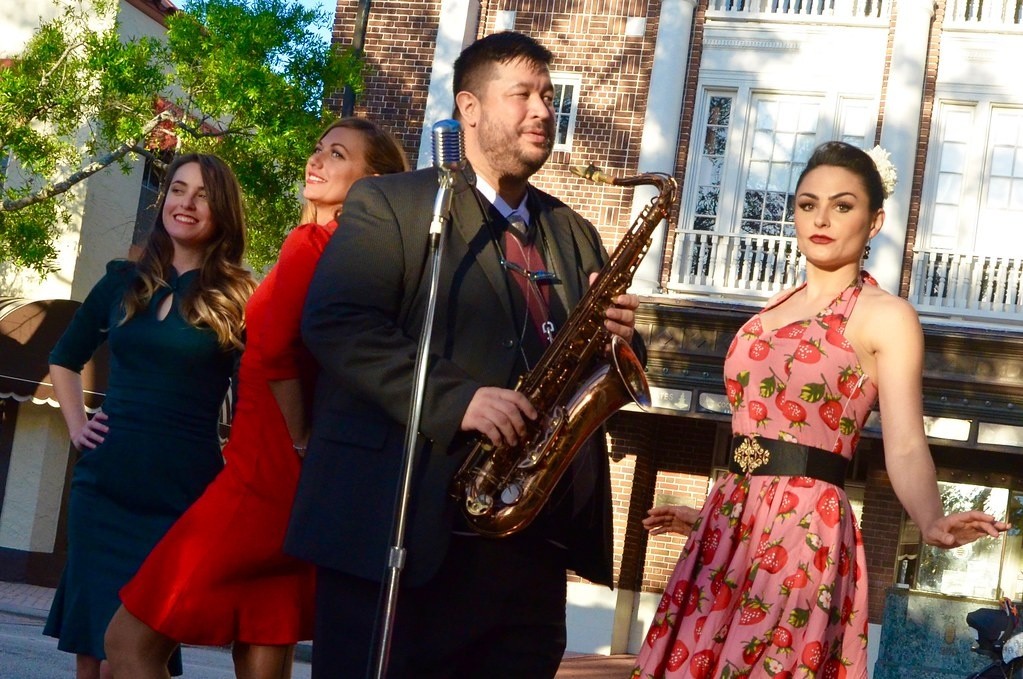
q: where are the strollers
[964,597,1023,679]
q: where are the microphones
[429,119,476,252]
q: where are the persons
[967,597,1023,648]
[282,32,647,679]
[105,117,407,679]
[44,153,259,679]
[629,141,1012,679]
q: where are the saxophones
[442,161,684,540]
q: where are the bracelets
[293,445,307,450]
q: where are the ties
[509,210,527,233]
